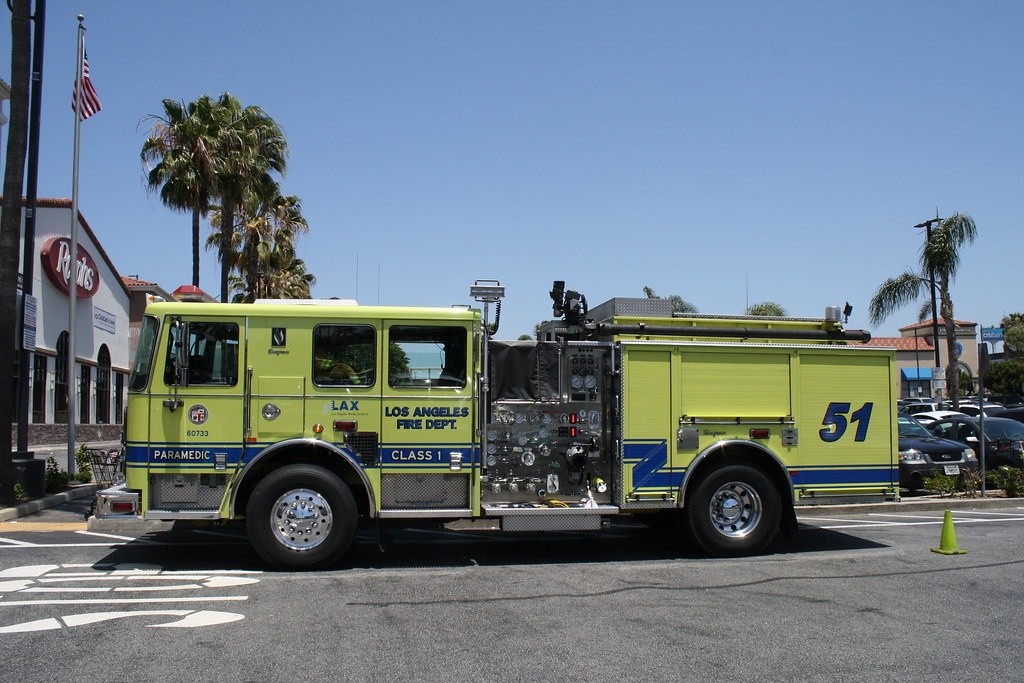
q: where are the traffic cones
[930,509,968,554]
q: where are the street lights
[914,218,946,412]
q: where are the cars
[897,395,1024,443]
[896,410,981,498]
[923,416,1024,489]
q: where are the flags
[70,25,101,121]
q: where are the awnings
[902,367,932,379]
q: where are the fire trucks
[92,279,903,572]
